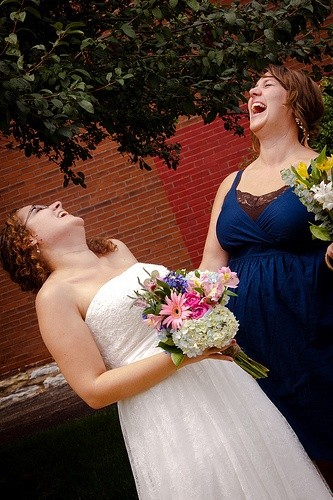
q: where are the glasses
[23,205,49,226]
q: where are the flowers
[279,144,332,270]
[124,266,270,380]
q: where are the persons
[196,68,333,486]
[0,200,333,500]
